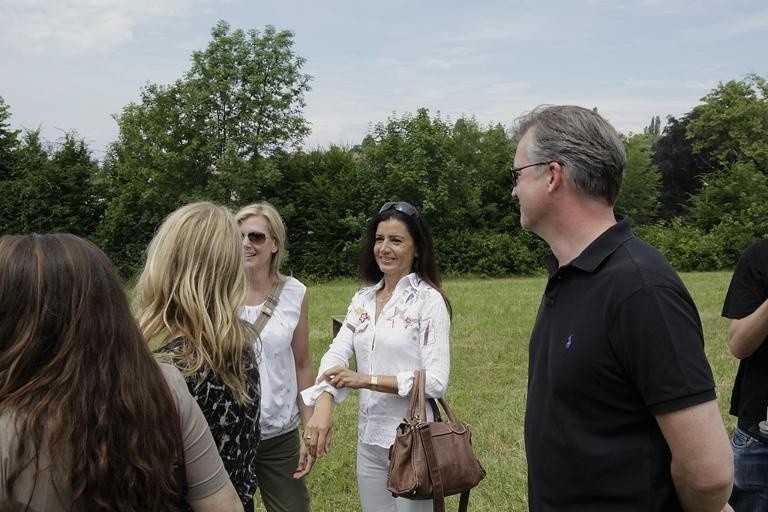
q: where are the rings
[306,436,311,440]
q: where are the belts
[737,418,767,444]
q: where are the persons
[511,102,733,512]
[234,204,316,512]
[1,232,248,510]
[719,239,766,511]
[297,201,454,512]
[129,201,260,512]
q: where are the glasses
[510,160,565,187]
[241,232,275,245]
[379,202,420,217]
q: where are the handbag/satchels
[386,370,486,501]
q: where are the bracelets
[370,374,377,391]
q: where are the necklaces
[377,293,392,302]
